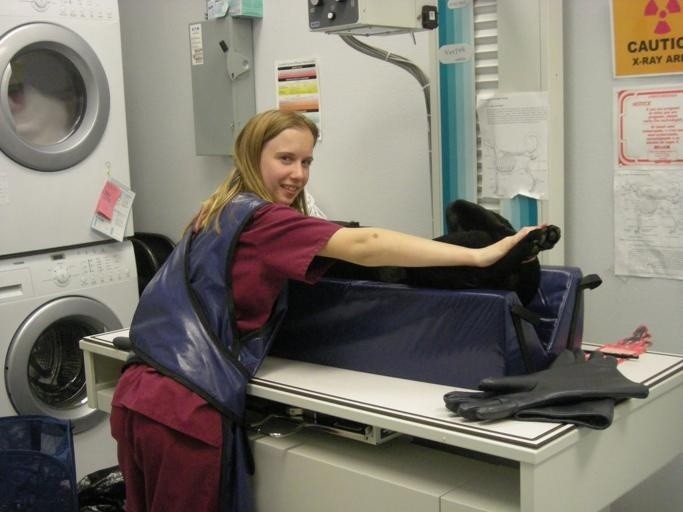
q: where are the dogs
[325,200,561,307]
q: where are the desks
[78,325,683,512]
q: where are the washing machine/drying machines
[0,240,141,490]
[0,2,136,259]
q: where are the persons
[109,108,544,512]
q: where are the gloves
[443,348,648,429]
